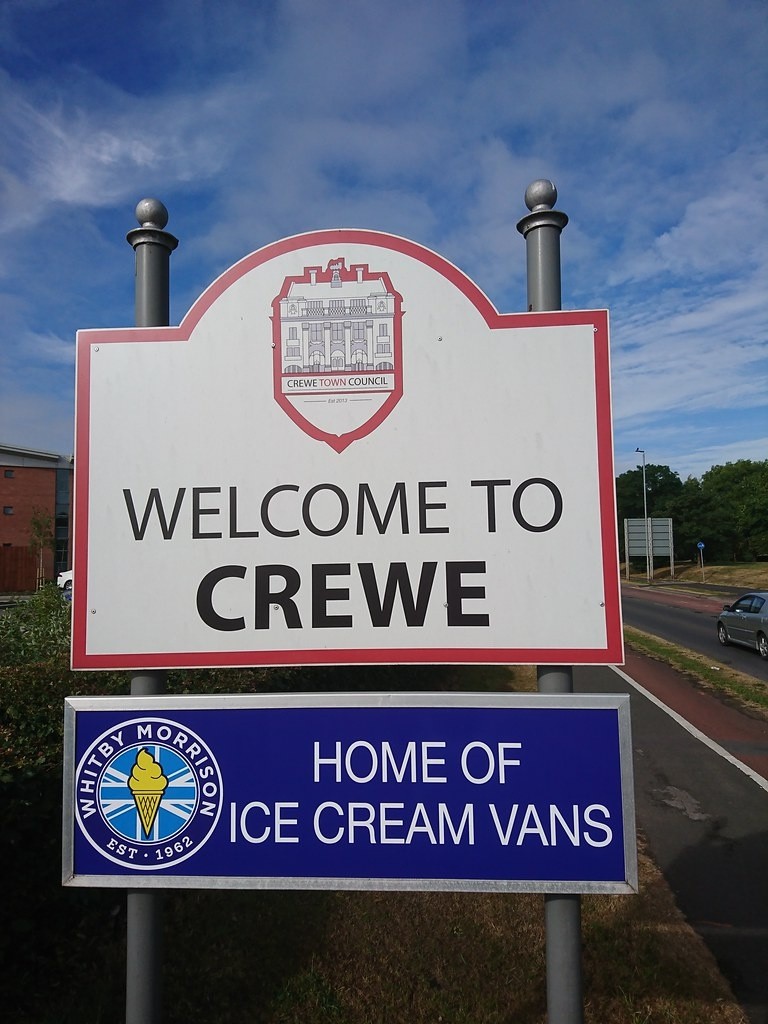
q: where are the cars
[57,569,72,590]
[716,593,767,657]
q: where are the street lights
[635,448,650,583]
